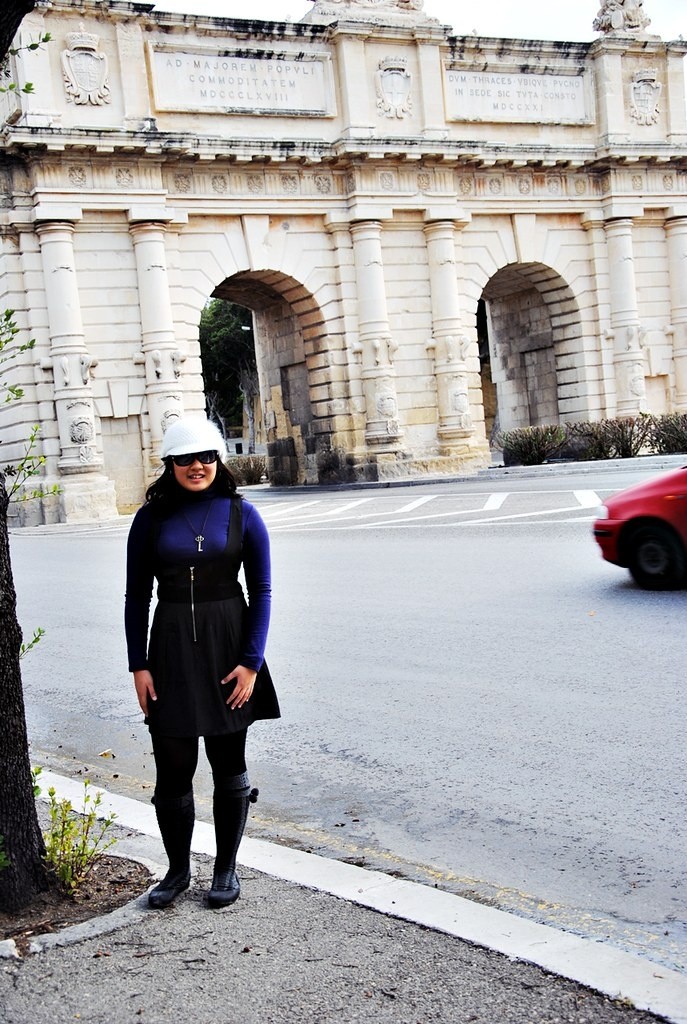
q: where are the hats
[160,416,226,463]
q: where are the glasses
[171,450,218,466]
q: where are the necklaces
[183,501,214,552]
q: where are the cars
[592,463,687,595]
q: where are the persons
[124,416,280,909]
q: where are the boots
[208,773,258,908]
[148,791,195,908]
